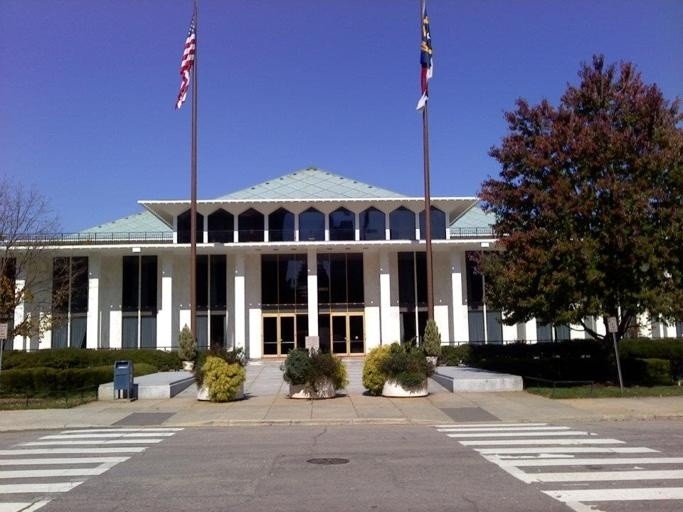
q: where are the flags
[415,3,433,111]
[174,8,196,112]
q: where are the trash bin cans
[114,360,133,399]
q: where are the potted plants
[176,323,198,372]
[420,318,442,367]
[194,341,435,403]
